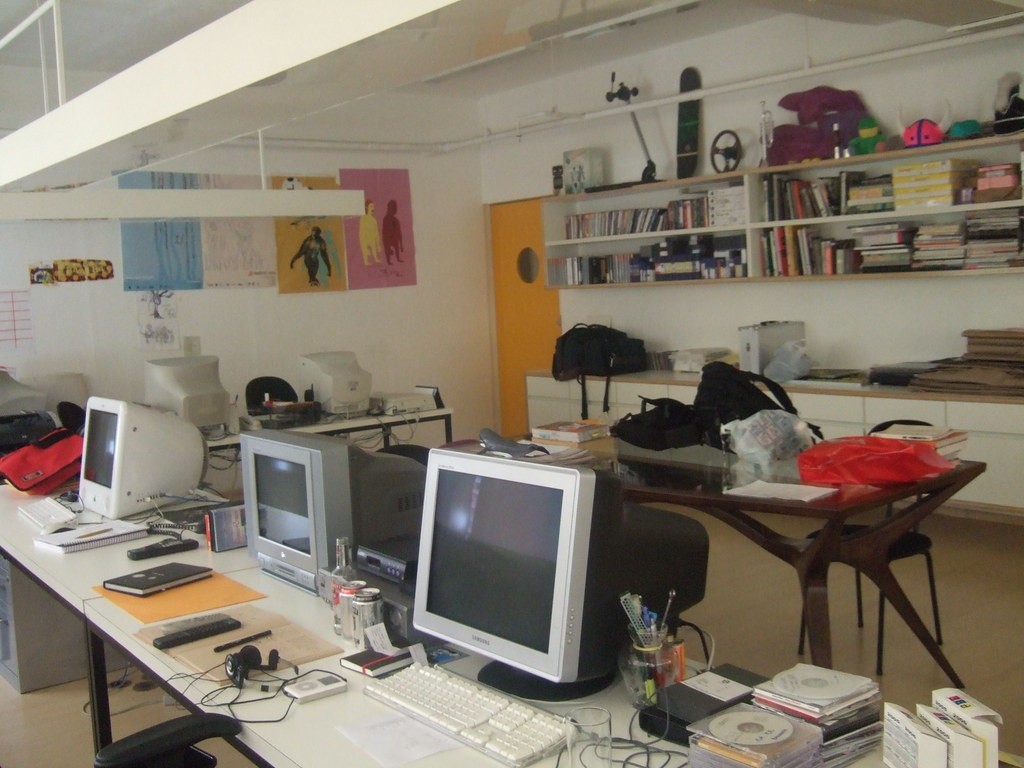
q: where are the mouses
[61,489,79,502]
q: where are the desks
[454,434,988,689]
[203,407,454,452]
[0,480,1023,768]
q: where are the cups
[565,707,612,768]
[672,639,685,682]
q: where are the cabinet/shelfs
[541,134,1024,292]
[525,376,1024,529]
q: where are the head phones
[225,645,299,693]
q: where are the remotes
[153,619,241,649]
[127,539,199,561]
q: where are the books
[531,420,610,443]
[102,562,213,596]
[34,519,151,554]
[871,423,970,470]
[547,157,1024,282]
[340,638,428,678]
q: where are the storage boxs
[893,158,982,211]
[564,147,604,195]
[882,687,1002,768]
[738,320,805,374]
[978,162,1018,189]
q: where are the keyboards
[362,660,579,768]
[18,497,79,535]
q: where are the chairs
[473,428,709,664]
[798,420,943,676]
[376,445,430,466]
[94,712,242,768]
[244,377,298,416]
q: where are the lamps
[0,0,366,221]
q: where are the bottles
[617,621,679,711]
[331,537,357,636]
[833,123,840,159]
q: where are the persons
[570,165,577,189]
[578,164,585,189]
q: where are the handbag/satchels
[615,361,954,493]
[551,323,648,381]
[0,426,84,496]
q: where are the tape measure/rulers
[620,594,651,646]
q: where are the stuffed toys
[850,115,886,156]
[895,101,950,147]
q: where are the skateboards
[676,68,701,179]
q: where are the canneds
[340,580,384,650]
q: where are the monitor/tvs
[241,429,428,596]
[412,445,709,702]
[145,358,230,440]
[298,351,373,419]
[78,396,210,521]
[0,370,47,419]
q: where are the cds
[708,711,793,745]
[774,667,854,699]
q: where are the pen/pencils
[213,629,273,654]
[640,605,664,688]
[78,525,112,539]
